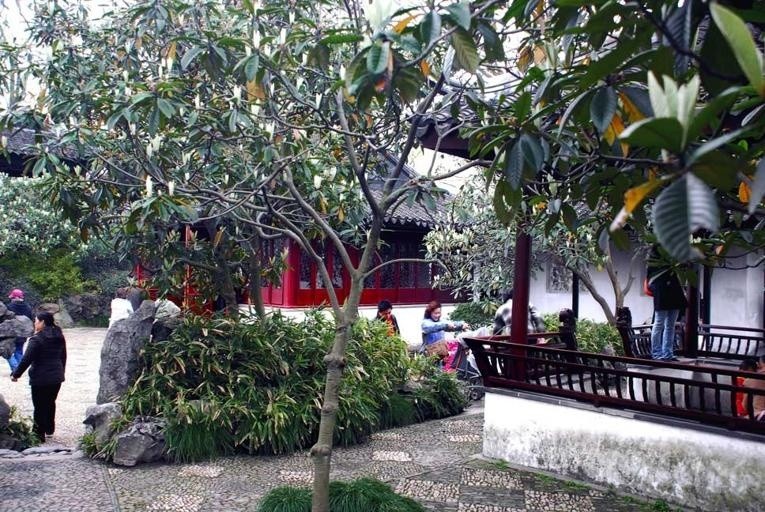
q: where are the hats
[9,289,24,299]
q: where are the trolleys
[441,323,492,402]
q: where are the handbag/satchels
[425,337,449,365]
[644,278,654,295]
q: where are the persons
[127,285,147,311]
[10,312,66,443]
[6,289,33,372]
[373,300,400,335]
[495,289,537,369]
[421,301,468,366]
[735,358,758,416]
[742,355,765,421]
[108,288,134,329]
[647,244,688,362]
